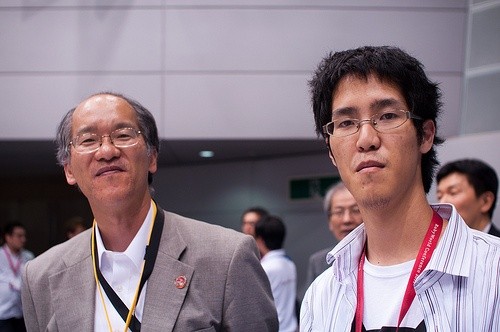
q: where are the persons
[299,46,500,332]
[0,223,34,332]
[242,207,298,332]
[307,182,363,288]
[20,92,279,332]
[436,159,500,238]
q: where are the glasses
[322,110,424,138]
[67,127,143,154]
